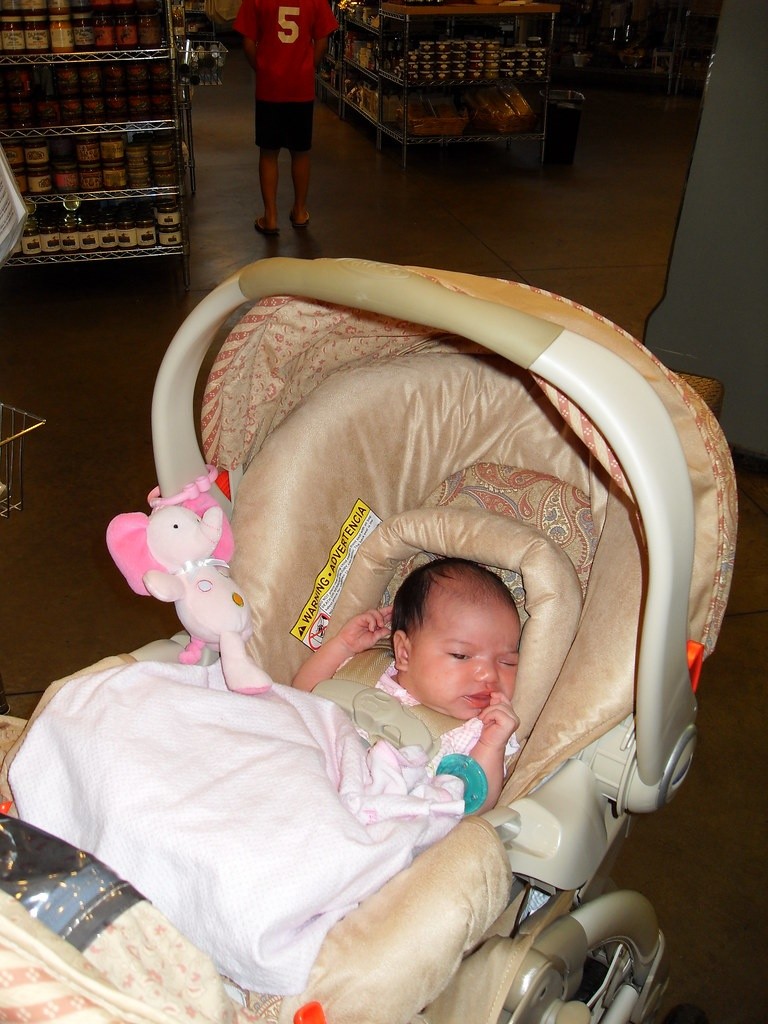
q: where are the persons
[289,555,521,817]
[233,0,338,234]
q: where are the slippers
[254,217,280,235]
[289,207,310,227]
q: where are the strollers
[5,256,738,1024]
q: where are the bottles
[189,44,224,86]
[330,69,339,90]
[366,43,374,71]
[356,2,372,23]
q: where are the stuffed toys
[106,492,275,694]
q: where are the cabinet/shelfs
[552,0,723,95]
[0,0,215,292]
[314,0,560,170]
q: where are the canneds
[0,0,163,55]
[12,197,182,256]
[0,60,174,132]
[1,130,178,195]
